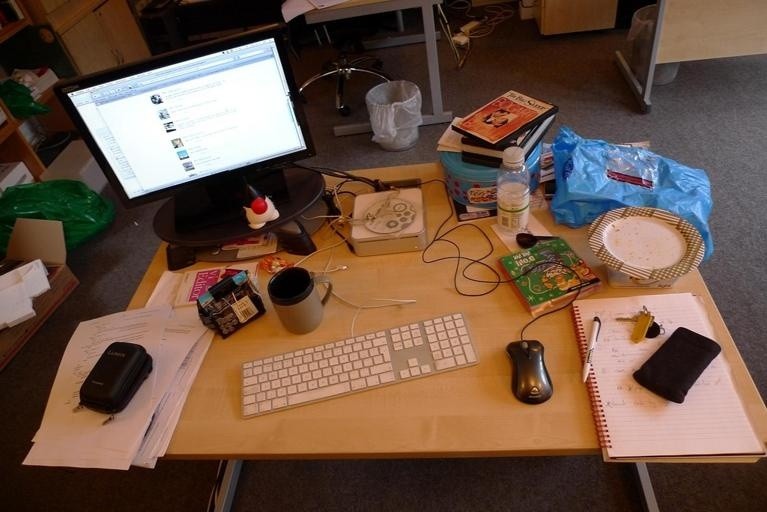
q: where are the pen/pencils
[582,317,602,384]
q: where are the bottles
[496,145,530,236]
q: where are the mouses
[505,339,557,406]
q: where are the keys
[616,305,665,346]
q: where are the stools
[297,18,393,116]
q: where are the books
[434,89,559,169]
[497,240,602,317]
[569,292,766,464]
[172,263,260,307]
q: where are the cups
[267,266,333,335]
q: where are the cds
[362,197,417,235]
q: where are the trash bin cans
[366,80,422,152]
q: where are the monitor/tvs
[48,22,326,247]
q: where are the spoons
[516,232,560,249]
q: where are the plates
[588,206,706,282]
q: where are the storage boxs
[0,218,80,372]
[39,140,108,195]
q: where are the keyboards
[240,312,480,416]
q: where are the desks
[124,163,767,511]
[304,1,452,137]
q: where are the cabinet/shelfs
[0,0,70,183]
[535,0,618,35]
[23,0,152,78]
